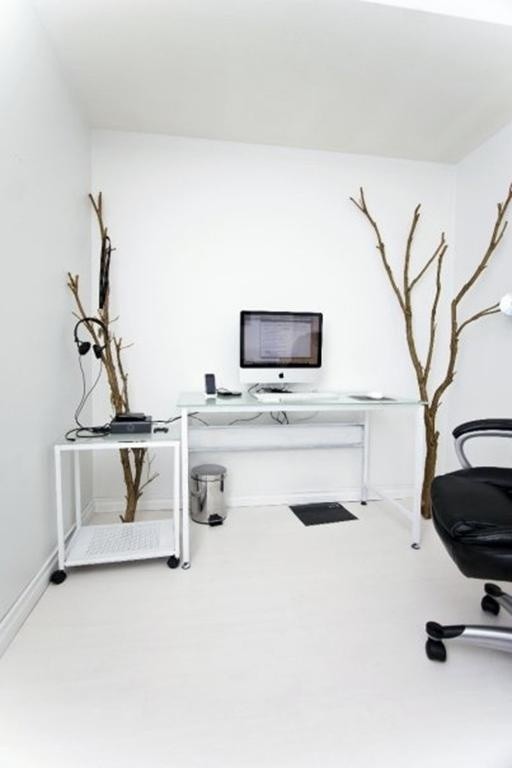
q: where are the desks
[51,432,181,585]
[177,391,427,570]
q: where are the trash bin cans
[190,463,229,526]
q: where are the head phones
[74,317,109,358]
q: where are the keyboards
[253,392,320,403]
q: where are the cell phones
[205,374,215,395]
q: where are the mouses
[366,391,385,400]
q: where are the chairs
[425,418,512,662]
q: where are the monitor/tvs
[240,310,323,384]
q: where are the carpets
[289,502,358,527]
